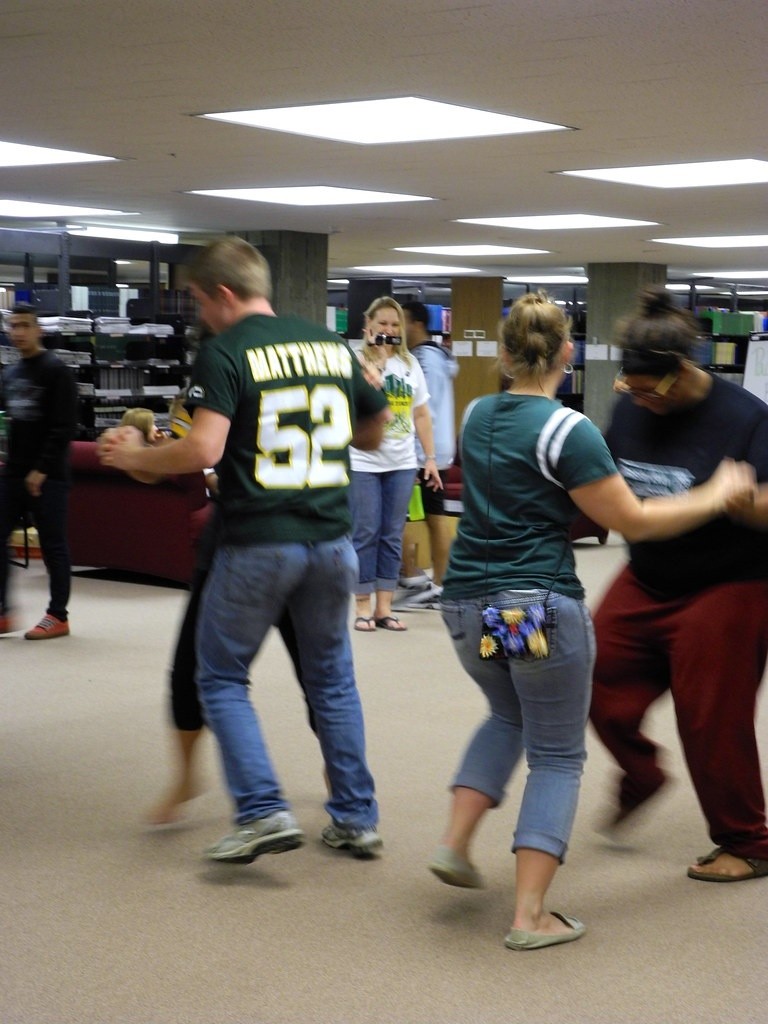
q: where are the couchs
[441,439,610,544]
[64,435,213,588]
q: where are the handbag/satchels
[479,601,549,662]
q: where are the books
[694,306,767,389]
[556,338,587,394]
[421,304,452,350]
[2,283,203,420]
[323,304,349,336]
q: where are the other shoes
[504,911,586,950]
[612,776,665,827]
[430,846,487,889]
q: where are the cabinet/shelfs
[0,227,193,440]
[328,278,768,425]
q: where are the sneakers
[321,823,383,852]
[391,573,430,604]
[25,614,70,639]
[203,811,305,864]
[404,580,443,610]
[0,614,22,633]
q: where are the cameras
[374,332,402,346]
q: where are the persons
[201,464,221,498]
[587,289,768,882]
[428,285,757,952]
[350,295,445,632]
[96,321,332,829]
[388,300,458,611]
[120,408,171,445]
[97,236,395,867]
[0,300,75,641]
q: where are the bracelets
[424,453,437,461]
[375,364,387,374]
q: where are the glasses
[615,371,677,402]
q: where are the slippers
[370,615,406,631]
[687,847,768,880]
[354,616,376,632]
